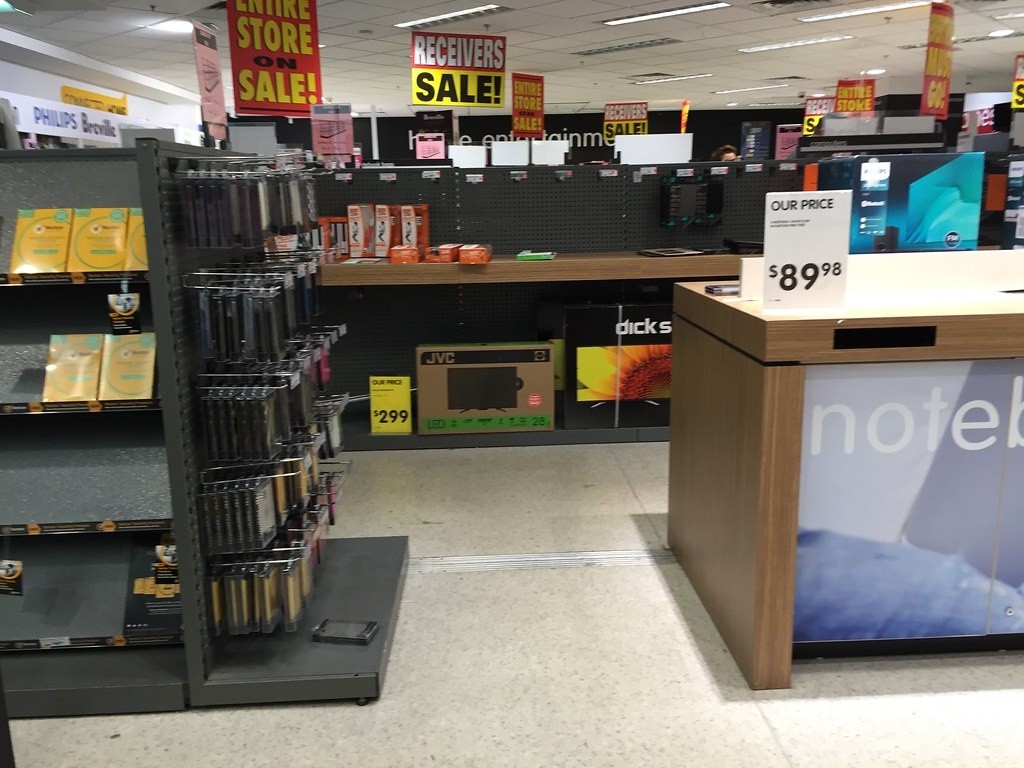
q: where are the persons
[712,145,737,161]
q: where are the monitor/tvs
[562,302,673,401]
[448,367,517,409]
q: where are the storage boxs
[401,204,430,254]
[424,243,463,264]
[347,203,375,258]
[41,334,105,406]
[315,216,330,254]
[415,341,554,436]
[458,244,492,264]
[374,205,402,258]
[816,151,986,254]
[98,332,158,403]
[327,216,350,255]
[391,245,426,264]
[9,208,74,275]
[66,208,130,275]
[123,207,149,271]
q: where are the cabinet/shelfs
[0,97,1024,718]
